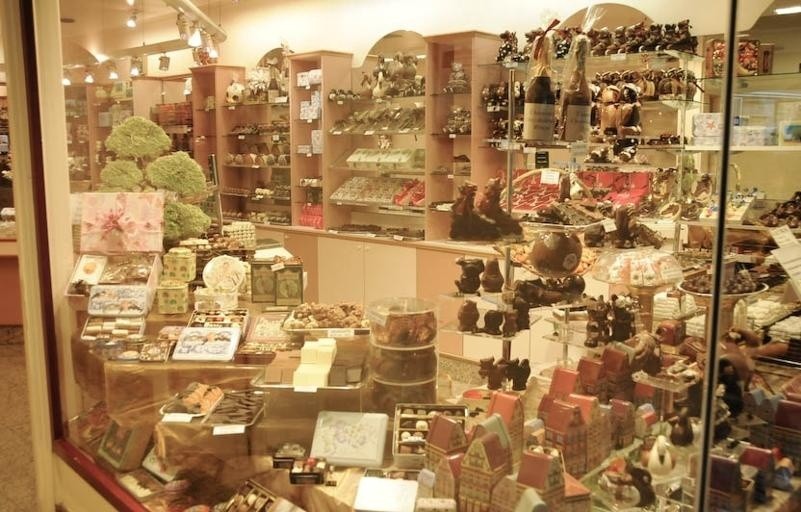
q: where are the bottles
[563,34,592,144]
[521,33,555,142]
